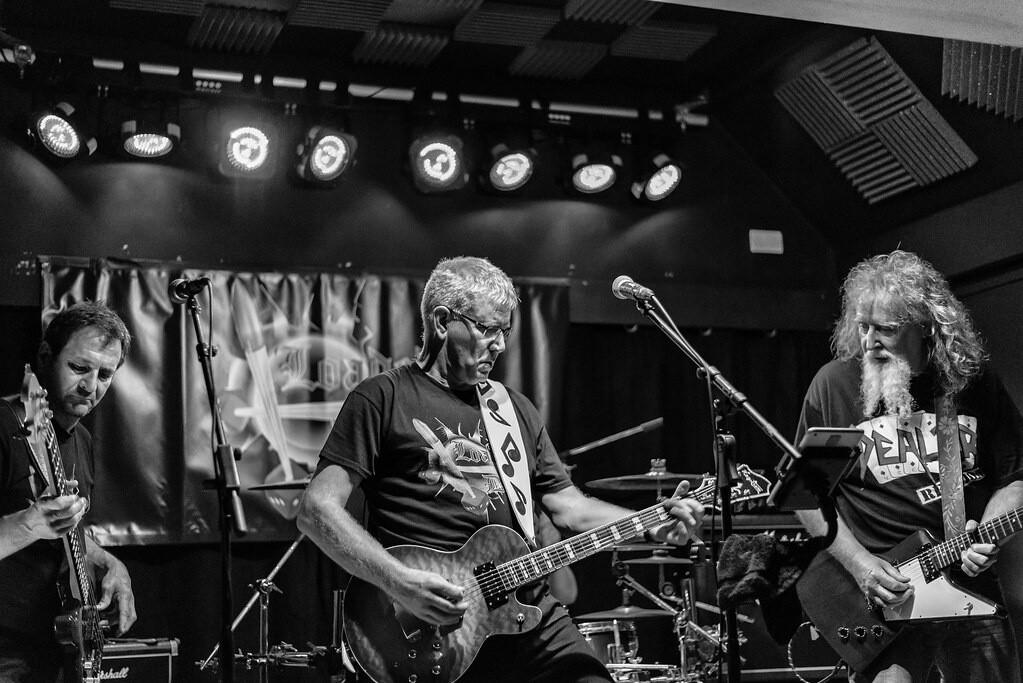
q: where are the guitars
[340,462,773,683]
[18,368,108,683]
[795,505,1023,676]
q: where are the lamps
[409,131,470,192]
[121,119,181,158]
[292,123,360,184]
[28,100,98,162]
[570,152,622,194]
[630,158,685,203]
[487,148,534,191]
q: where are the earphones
[440,316,447,331]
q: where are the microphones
[168,276,209,304]
[612,275,655,301]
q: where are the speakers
[98,637,179,683]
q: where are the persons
[0,303,136,683]
[295,258,704,682]
[787,248,1023,683]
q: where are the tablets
[767,428,864,507]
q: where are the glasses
[450,309,514,342]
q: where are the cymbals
[584,455,713,492]
[619,549,694,565]
[601,543,678,551]
[574,602,675,619]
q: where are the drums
[575,621,637,674]
